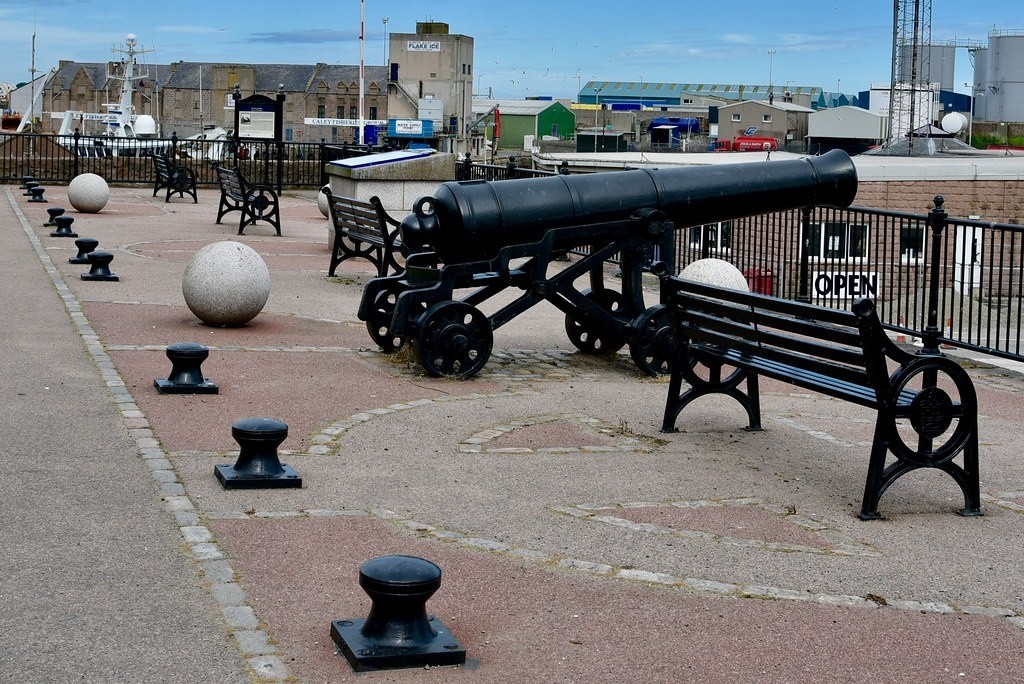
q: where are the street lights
[593,87,602,152]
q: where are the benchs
[149,150,199,204]
[212,161,282,236]
[651,260,986,520]
[322,186,437,278]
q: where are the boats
[51,33,225,158]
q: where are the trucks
[714,136,778,153]
